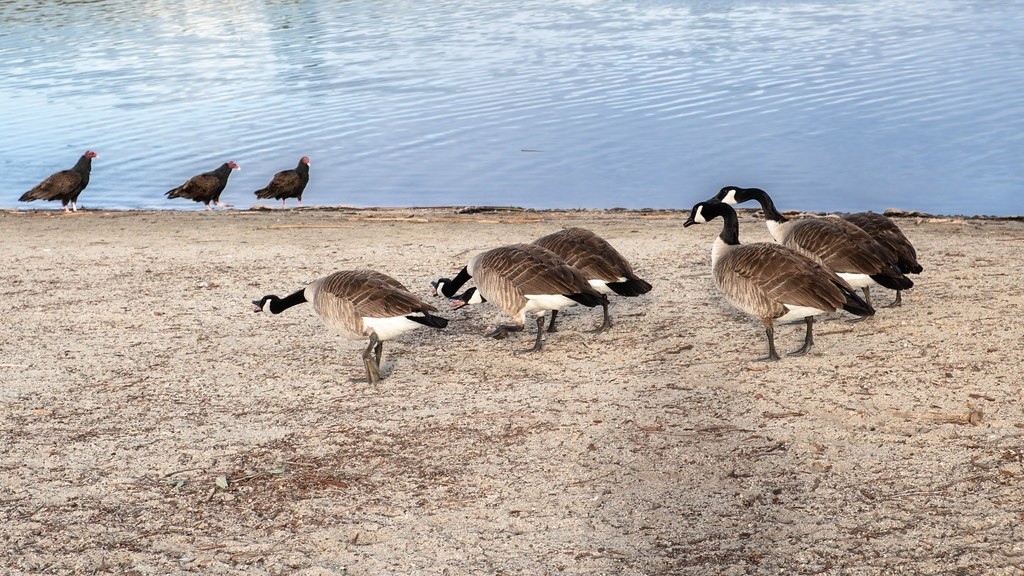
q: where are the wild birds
[683,201,877,363]
[842,212,923,308]
[708,185,914,324]
[431,243,610,354]
[251,269,451,386]
[451,226,652,333]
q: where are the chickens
[18,149,99,213]
[254,156,310,210]
[163,161,240,212]
